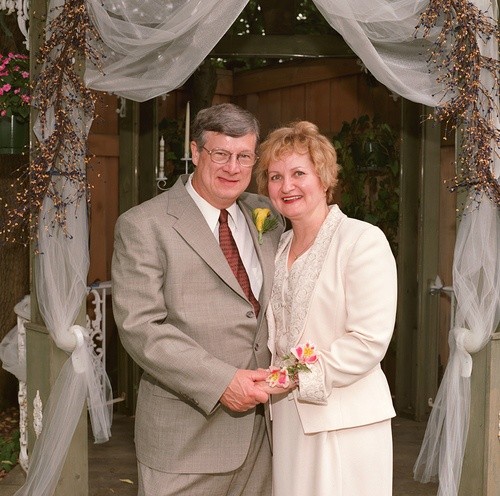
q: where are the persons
[250,121,397,496]
[112,103,283,496]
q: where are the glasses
[202,146,259,166]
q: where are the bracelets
[267,363,300,391]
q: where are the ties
[219,209,261,320]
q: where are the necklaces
[292,236,316,262]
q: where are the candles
[158,134,166,177]
[184,101,191,159]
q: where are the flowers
[0,51,30,128]
[251,207,279,244]
